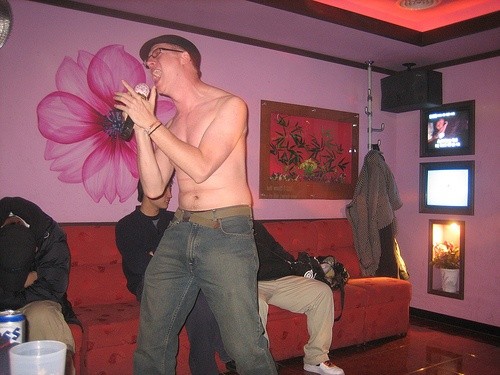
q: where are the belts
[174,205,251,229]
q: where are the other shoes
[226,360,237,370]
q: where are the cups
[9,340,66,375]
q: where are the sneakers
[304,361,344,375]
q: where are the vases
[442,269,460,293]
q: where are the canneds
[0,310,26,346]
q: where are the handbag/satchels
[294,252,350,291]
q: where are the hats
[140,34,201,70]
[0,222,36,291]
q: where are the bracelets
[147,119,160,133]
[148,123,162,136]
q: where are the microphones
[122,82,150,142]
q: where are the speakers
[381,69,442,113]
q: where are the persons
[0,197,76,375]
[113,34,278,375]
[253,219,344,375]
[429,118,456,148]
[116,168,237,375]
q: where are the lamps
[399,0,441,11]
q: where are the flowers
[433,241,461,269]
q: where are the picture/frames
[259,100,359,199]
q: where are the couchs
[57,218,413,375]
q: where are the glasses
[143,47,183,69]
